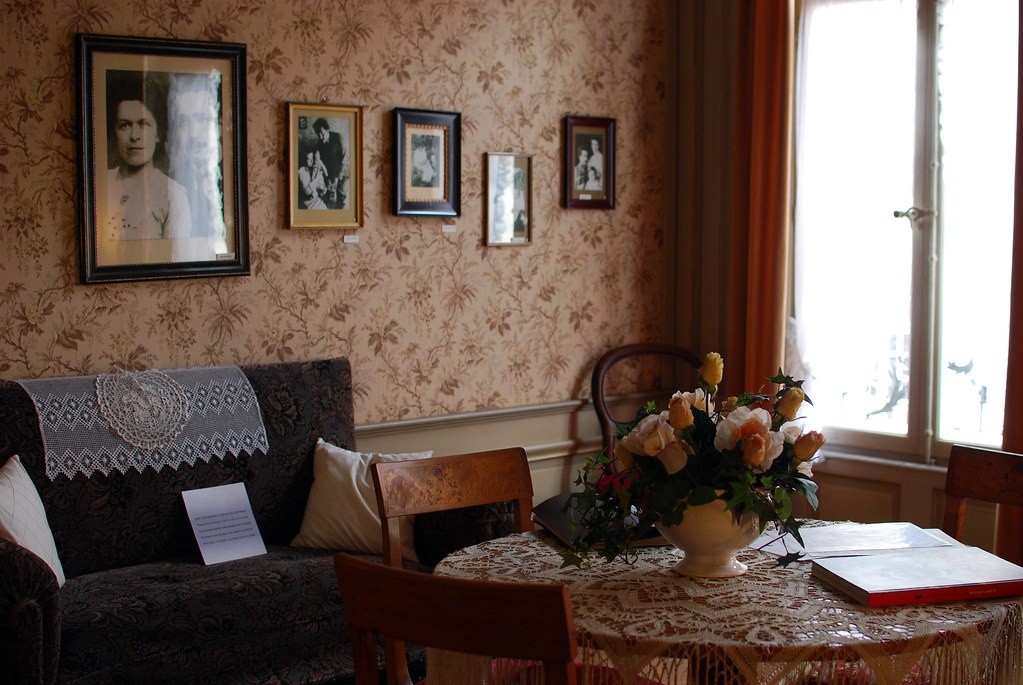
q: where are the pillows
[289,438,434,554]
[1,454,68,589]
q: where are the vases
[655,490,767,579]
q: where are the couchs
[0,354,519,685]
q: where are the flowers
[557,351,825,572]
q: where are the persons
[109,95,607,245]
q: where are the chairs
[335,549,580,685]
[946,442,1023,566]
[591,344,705,480]
[370,445,538,683]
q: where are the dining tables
[430,519,1023,685]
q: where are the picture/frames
[559,115,617,210]
[390,108,464,219]
[72,31,253,285]
[284,100,367,233]
[481,151,534,247]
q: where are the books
[810,546,1023,608]
[531,493,673,553]
